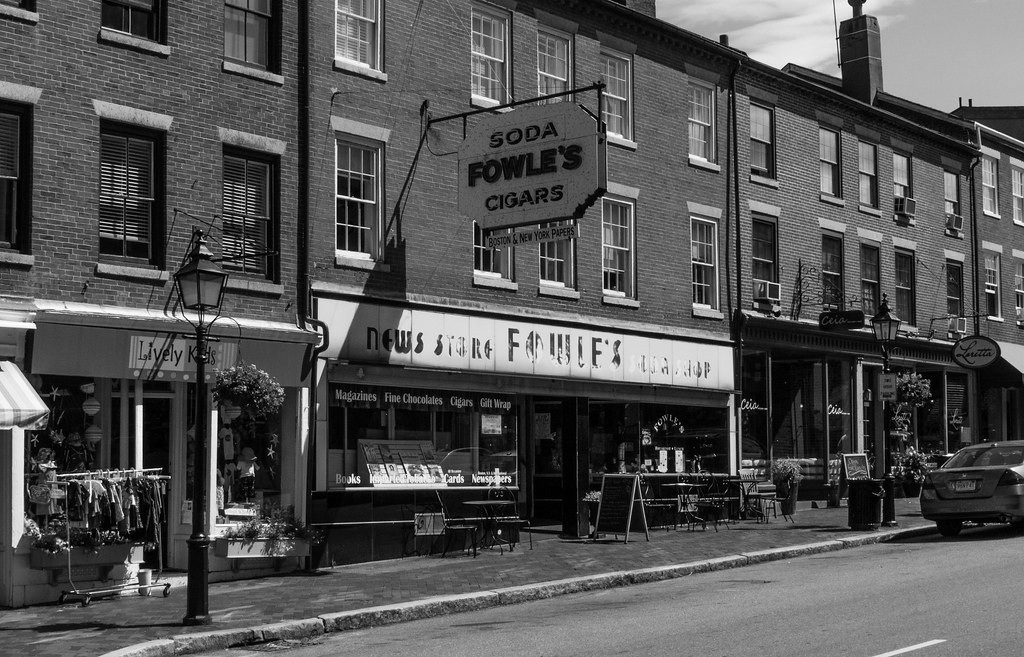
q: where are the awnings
[986,341,1024,389]
[0,361,50,430]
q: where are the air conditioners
[948,318,967,334]
[946,213,964,232]
[893,196,916,218]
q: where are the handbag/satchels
[29,484,50,504]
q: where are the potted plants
[900,446,930,499]
[215,499,325,559]
[30,525,146,570]
[768,455,805,514]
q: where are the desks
[581,499,619,540]
[722,478,766,523]
[217,508,256,524]
[659,481,707,533]
[461,499,515,558]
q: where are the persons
[237,447,259,498]
[35,432,87,516]
[838,428,852,453]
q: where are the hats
[240,447,257,460]
[35,448,54,460]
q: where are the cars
[432,440,498,474]
[920,440,1024,536]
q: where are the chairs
[642,476,675,531]
[488,485,534,555]
[678,468,718,533]
[740,467,777,519]
[435,491,479,559]
[696,470,729,531]
[765,472,796,525]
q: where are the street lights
[172,229,230,626]
[867,291,902,528]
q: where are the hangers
[68,466,160,480]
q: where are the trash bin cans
[847,479,884,532]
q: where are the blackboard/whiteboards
[595,473,648,535]
[839,454,870,500]
